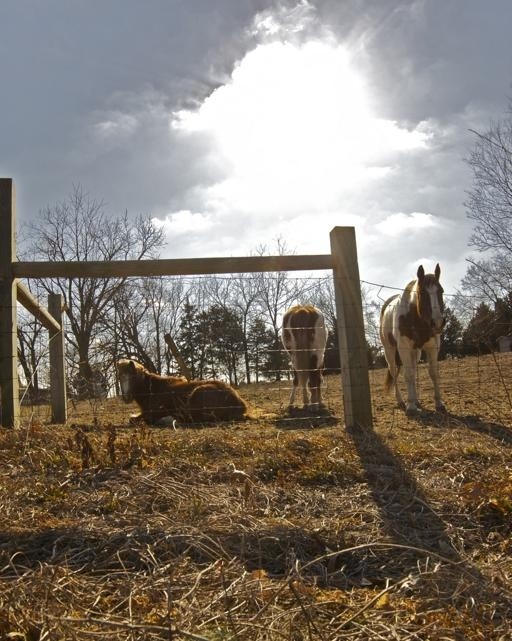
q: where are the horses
[379,263,444,416]
[115,358,246,426]
[280,304,328,419]
